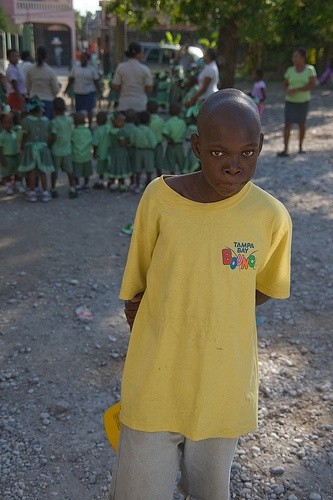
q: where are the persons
[131,112,158,195]
[72,108,94,194]
[182,104,204,177]
[48,97,78,201]
[0,109,19,195]
[21,100,55,201]
[17,50,33,97]
[191,47,220,112]
[112,88,293,500]
[107,72,121,109]
[62,73,76,111]
[75,33,111,68]
[5,49,25,97]
[247,68,266,125]
[51,35,63,68]
[114,111,131,194]
[112,41,154,127]
[63,50,100,128]
[147,100,165,179]
[145,52,199,115]
[96,70,105,102]
[26,45,63,120]
[91,111,110,192]
[162,96,187,178]
[277,49,317,159]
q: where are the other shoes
[0,178,142,202]
